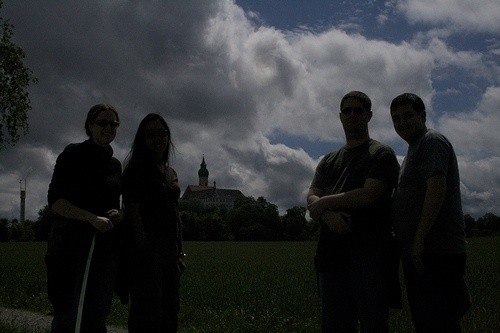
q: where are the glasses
[341,105,368,115]
[91,118,120,128]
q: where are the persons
[43,101,125,333]
[305,90,403,333]
[388,91,471,332]
[120,112,187,332]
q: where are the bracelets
[176,252,187,258]
